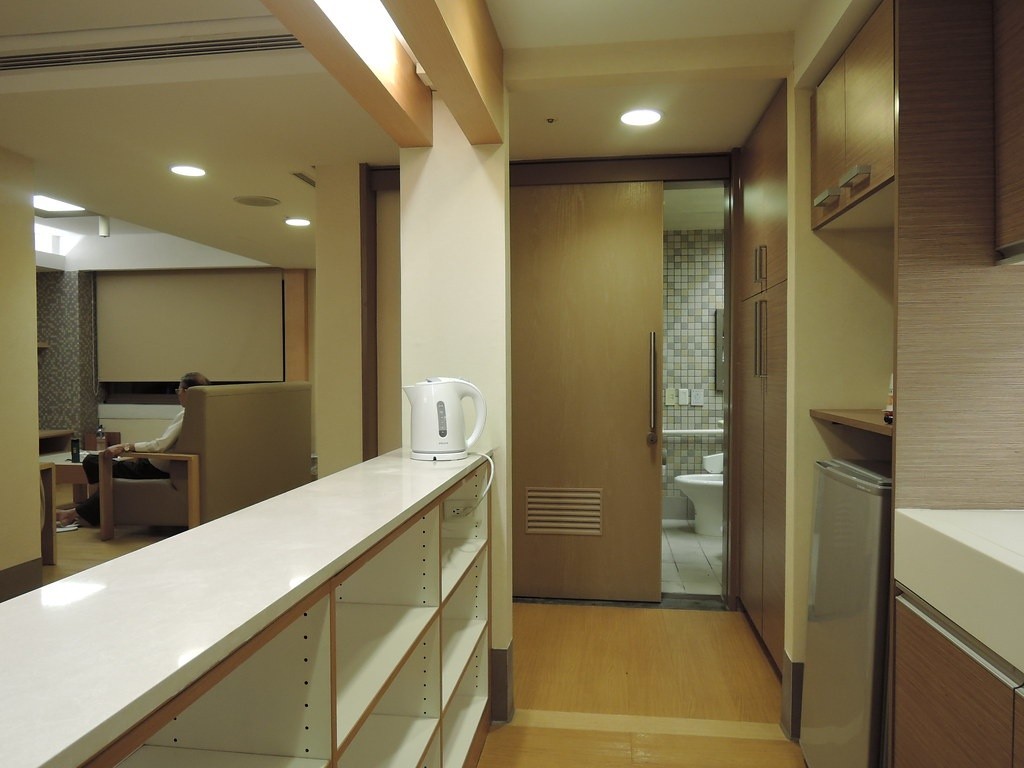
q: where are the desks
[38,450,94,506]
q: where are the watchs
[124,443,129,452]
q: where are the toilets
[674,473,723,537]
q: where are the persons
[57,373,209,527]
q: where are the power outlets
[444,500,473,520]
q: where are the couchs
[98,382,312,542]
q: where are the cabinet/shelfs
[734,78,783,676]
[0,447,494,768]
[808,0,1024,768]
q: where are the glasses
[175,389,185,395]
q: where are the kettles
[402,376,487,460]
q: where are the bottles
[888,374,893,409]
[96,425,105,450]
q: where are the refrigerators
[799,456,892,768]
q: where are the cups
[71,439,80,463]
